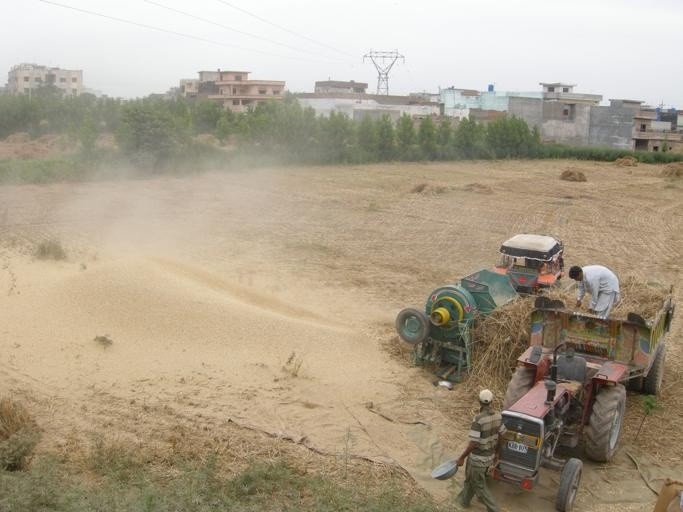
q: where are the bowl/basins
[430,460,459,482]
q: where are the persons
[455,389,508,512]
[569,265,620,329]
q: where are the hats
[479,390,493,404]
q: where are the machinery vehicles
[487,281,675,512]
